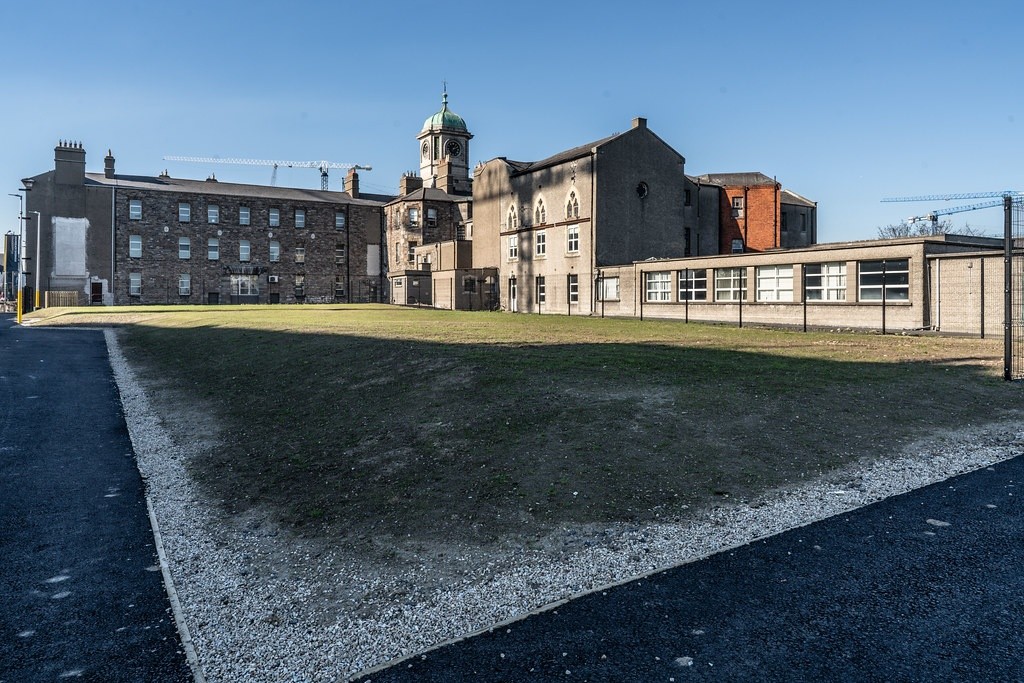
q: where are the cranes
[161,155,372,190]
[907,196,1024,236]
[880,191,1024,203]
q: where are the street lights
[8,193,23,325]
[28,210,41,309]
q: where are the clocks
[445,139,462,158]
[421,142,429,159]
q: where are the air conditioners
[269,276,279,283]
